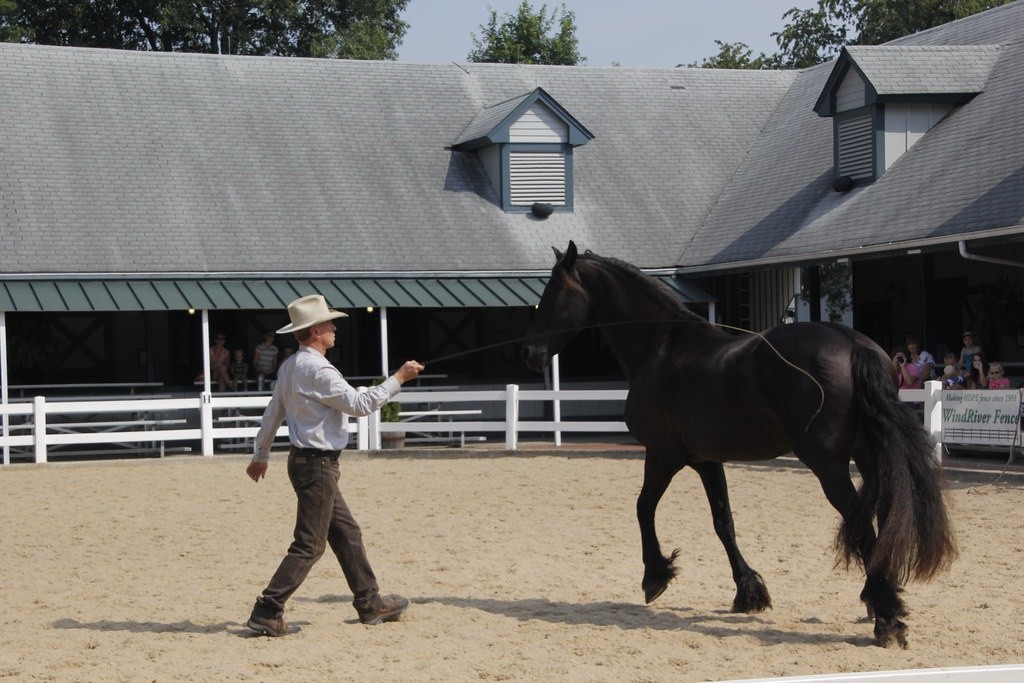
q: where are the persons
[938,352,971,390]
[246,294,425,636]
[890,346,922,389]
[211,333,232,392]
[958,331,982,389]
[230,349,248,392]
[253,331,279,391]
[987,362,1010,390]
[970,351,990,389]
[279,345,294,368]
[905,335,936,388]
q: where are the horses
[520,240,959,650]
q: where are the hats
[276,295,349,334]
[960,328,976,340]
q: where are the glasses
[990,371,1002,375]
[265,334,275,338]
[216,337,227,341]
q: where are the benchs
[8,382,192,460]
[193,373,487,448]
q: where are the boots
[352,594,410,625]
[247,596,302,637]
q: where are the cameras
[898,357,904,363]
